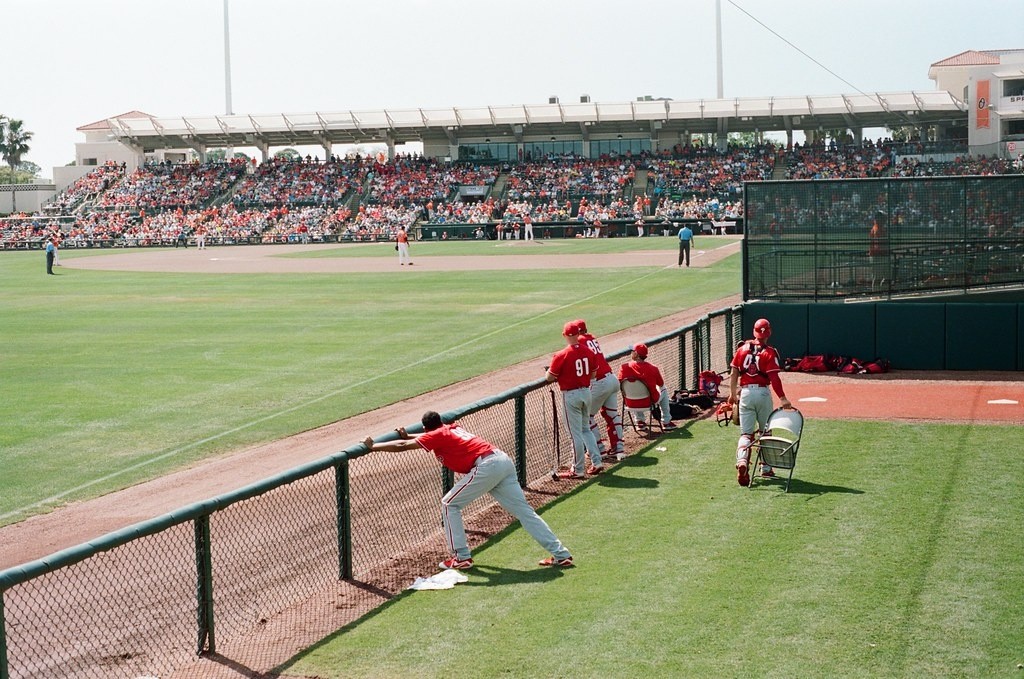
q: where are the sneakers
[439,556,474,570]
[559,469,584,478]
[585,451,607,460]
[662,421,677,430]
[607,449,625,460]
[736,464,750,486]
[762,468,776,477]
[636,422,647,430]
[587,466,605,475]
[537,555,573,567]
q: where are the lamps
[988,102,995,111]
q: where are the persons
[45,233,61,274]
[730,319,791,486]
[678,223,694,267]
[0,137,1024,292]
[544,322,603,478]
[358,410,573,569]
[620,343,677,431]
[573,320,624,458]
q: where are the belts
[744,384,767,388]
[568,386,588,391]
[473,450,493,467]
[596,372,612,381]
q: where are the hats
[564,322,579,337]
[573,320,586,332]
[633,344,649,356]
[753,318,771,339]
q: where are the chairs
[740,403,804,495]
[619,377,665,442]
